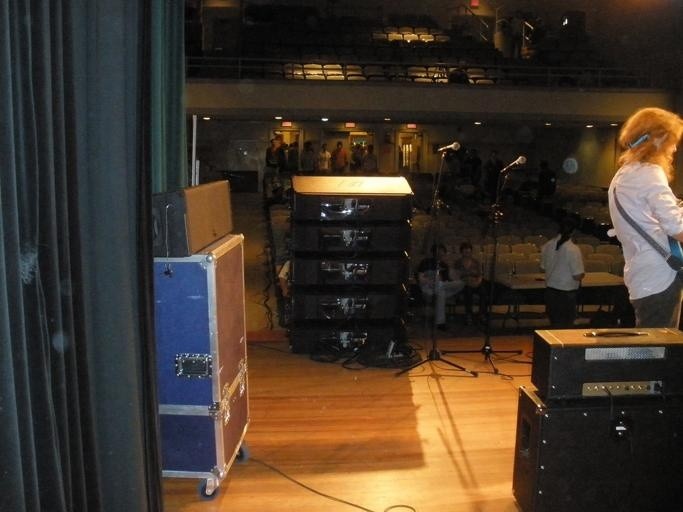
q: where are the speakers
[511,383,682,512]
[152,179,233,257]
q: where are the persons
[536,161,557,198]
[456,242,490,325]
[608,106,683,328]
[448,59,471,84]
[415,244,463,332]
[460,148,481,185]
[538,219,585,330]
[265,139,377,174]
[532,18,547,64]
[483,151,504,205]
[511,10,524,64]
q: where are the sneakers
[437,324,449,330]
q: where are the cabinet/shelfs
[285,174,411,357]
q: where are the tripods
[394,151,476,376]
[442,172,524,374]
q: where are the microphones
[437,142,460,152]
[500,155,526,173]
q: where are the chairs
[279,21,682,91]
[407,178,635,329]
